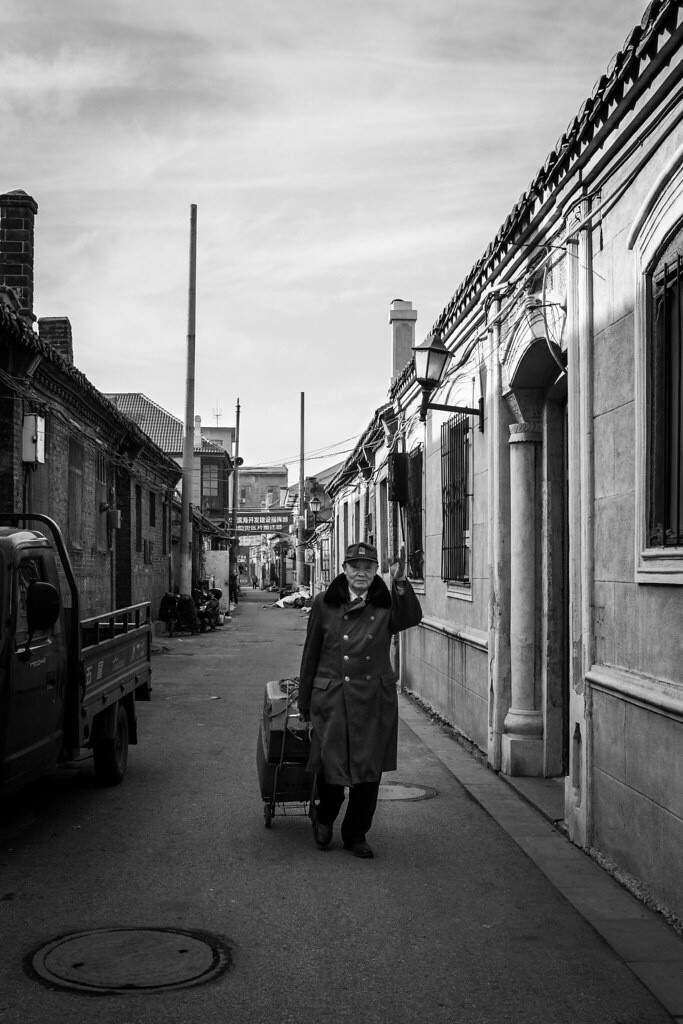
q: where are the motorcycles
[0,512,153,794]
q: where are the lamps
[308,494,335,530]
[410,327,485,433]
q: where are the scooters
[164,592,193,638]
[194,588,223,633]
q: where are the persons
[252,575,258,589]
[229,569,238,605]
[296,543,422,858]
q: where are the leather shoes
[344,834,373,857]
[315,814,332,845]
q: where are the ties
[355,597,363,603]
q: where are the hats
[344,542,379,563]
